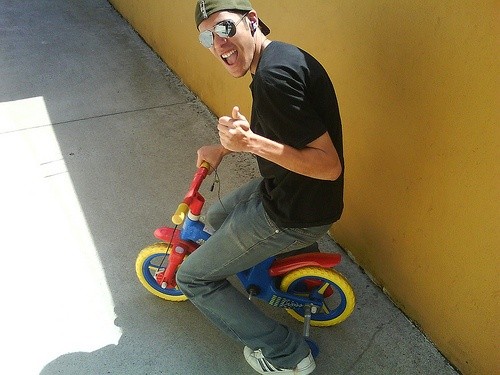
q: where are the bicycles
[131,160,357,359]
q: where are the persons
[173,1,346,375]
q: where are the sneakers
[244,346,316,375]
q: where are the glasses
[198,14,246,48]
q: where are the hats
[195,0,271,36]
